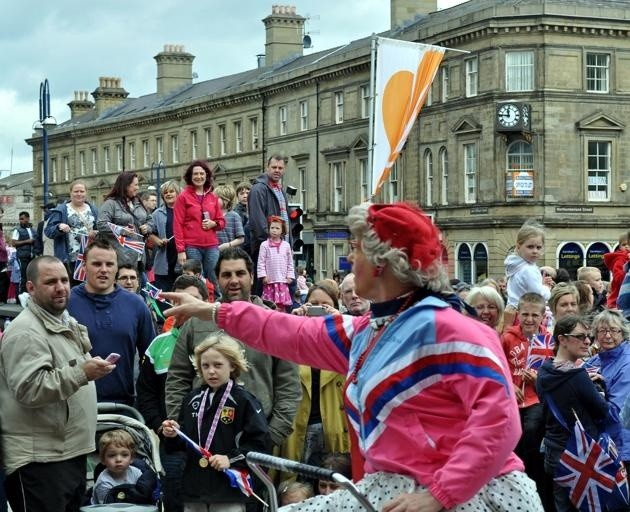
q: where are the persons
[0,153,630,512]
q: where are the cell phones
[103,353,122,366]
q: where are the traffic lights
[287,203,303,254]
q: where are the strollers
[80,402,166,512]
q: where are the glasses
[597,326,623,335]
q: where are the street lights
[148,161,168,208]
[32,79,56,210]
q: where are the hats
[367,205,442,271]
[562,332,595,342]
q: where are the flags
[371,36,448,203]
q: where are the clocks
[495,102,531,131]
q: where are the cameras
[307,306,328,317]
